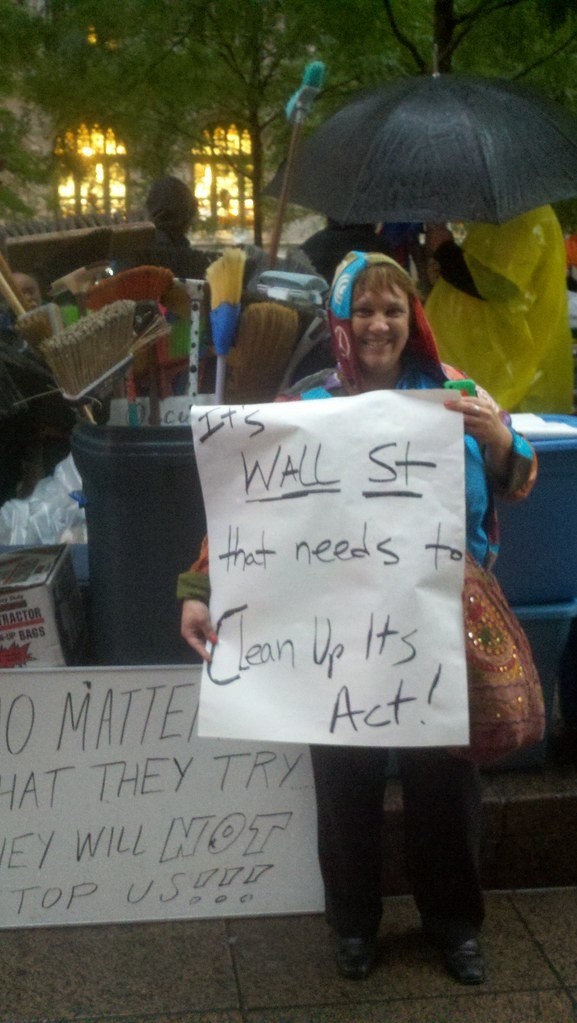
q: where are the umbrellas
[263,41,577,235]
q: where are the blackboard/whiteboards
[0,668,326,930]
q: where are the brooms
[203,246,300,405]
[13,264,207,425]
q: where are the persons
[177,250,537,984]
[422,205,574,415]
[0,272,44,330]
[299,215,404,287]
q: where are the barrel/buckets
[68,402,209,665]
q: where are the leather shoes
[443,937,485,984]
[336,928,373,979]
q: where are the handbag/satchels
[457,550,545,766]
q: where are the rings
[472,406,480,415]
